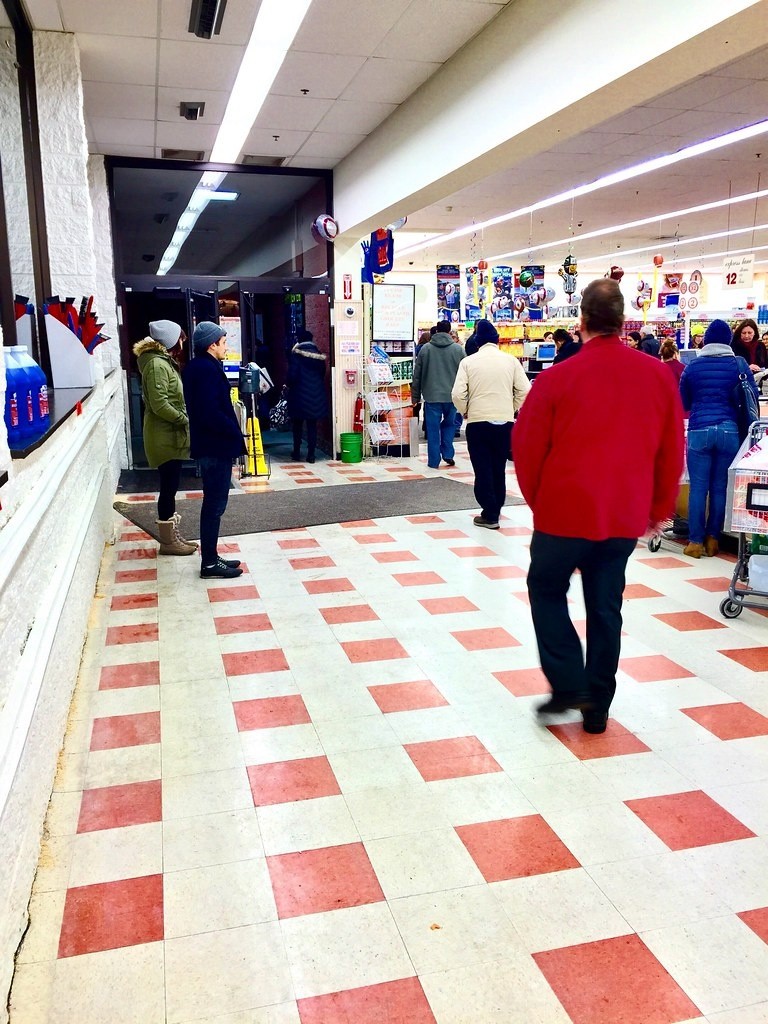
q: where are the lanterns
[653,254,664,268]
[478,259,488,271]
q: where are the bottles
[4,345,50,452]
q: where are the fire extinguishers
[353,393,364,433]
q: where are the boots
[155,512,199,556]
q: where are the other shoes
[580,710,609,733]
[306,456,315,463]
[536,687,592,714]
[442,458,455,466]
[290,452,300,461]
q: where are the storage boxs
[366,363,395,444]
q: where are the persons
[255,337,274,431]
[411,320,481,469]
[451,319,532,529]
[627,322,704,418]
[183,321,250,579]
[679,320,760,558]
[732,319,768,394]
[287,331,326,463]
[133,320,199,556]
[543,329,582,387]
[512,279,685,734]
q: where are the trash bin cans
[340,433,362,463]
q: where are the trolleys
[719,421,768,619]
[648,512,689,553]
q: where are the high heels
[683,541,703,558]
[706,535,720,557]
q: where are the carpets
[113,476,530,544]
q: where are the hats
[703,319,733,345]
[473,319,499,346]
[193,321,227,349]
[641,322,653,335]
[149,319,182,349]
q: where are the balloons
[310,214,337,242]
[444,256,681,320]
[384,217,407,232]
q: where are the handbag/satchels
[249,361,275,397]
[268,385,293,428]
[732,356,760,437]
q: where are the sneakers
[473,516,500,529]
[199,560,243,579]
[201,555,241,568]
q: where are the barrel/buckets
[340,432,362,463]
